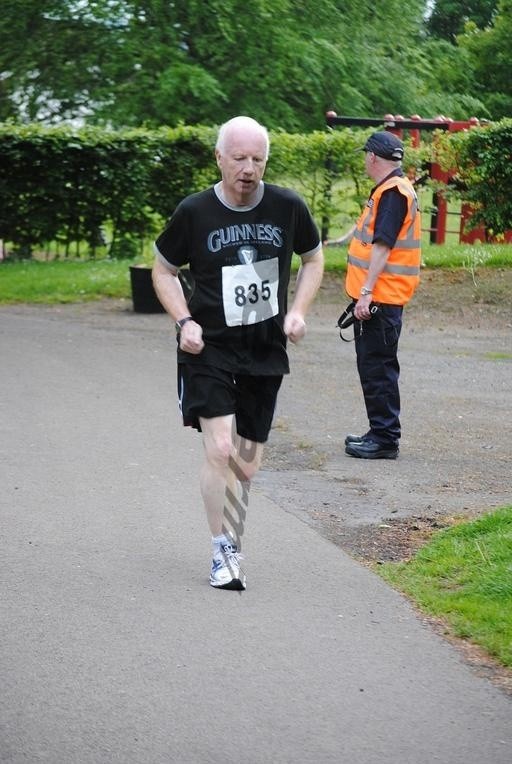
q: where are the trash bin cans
[129,266,167,313]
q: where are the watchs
[175,315,194,335]
[360,286,372,296]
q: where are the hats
[354,131,404,161]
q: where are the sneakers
[346,438,398,458]
[344,433,370,444]
[209,543,246,589]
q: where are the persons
[335,128,421,460]
[148,113,324,591]
